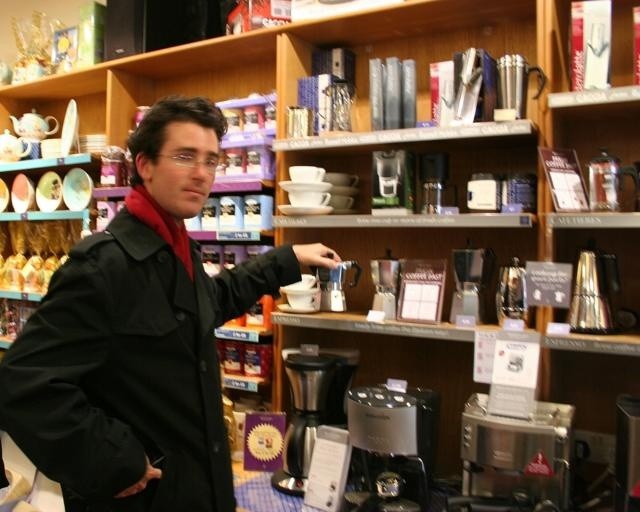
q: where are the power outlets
[571,429,617,464]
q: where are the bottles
[466,171,537,214]
[495,257,533,328]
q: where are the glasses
[154,153,223,175]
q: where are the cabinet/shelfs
[1,28,279,412]
[544,3,638,410]
[280,0,543,406]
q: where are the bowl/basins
[0,178,10,213]
[62,168,94,211]
[10,173,36,213]
[35,171,66,212]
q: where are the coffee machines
[270,352,353,497]
[343,385,447,512]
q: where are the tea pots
[568,237,621,335]
[0,130,31,164]
[9,109,60,140]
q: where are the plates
[278,303,318,313]
[279,271,316,293]
[61,97,108,158]
[277,205,354,216]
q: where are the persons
[0,94,342,512]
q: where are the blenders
[448,240,496,325]
[311,252,362,313]
[418,149,462,216]
[369,250,402,319]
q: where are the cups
[286,290,318,308]
[40,139,62,160]
[377,151,402,199]
[321,79,356,132]
[22,140,40,160]
[498,54,547,120]
[586,146,638,212]
[222,391,272,453]
[286,106,316,138]
[277,164,362,210]
